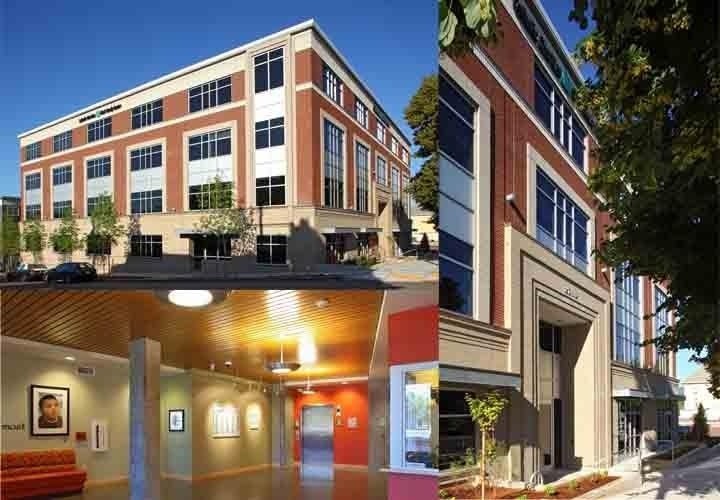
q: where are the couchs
[0,449,89,500]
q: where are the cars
[38,262,97,284]
[7,262,50,281]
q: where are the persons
[37,395,63,429]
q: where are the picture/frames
[169,409,184,431]
[30,384,69,437]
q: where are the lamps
[151,289,233,309]
[264,339,302,374]
